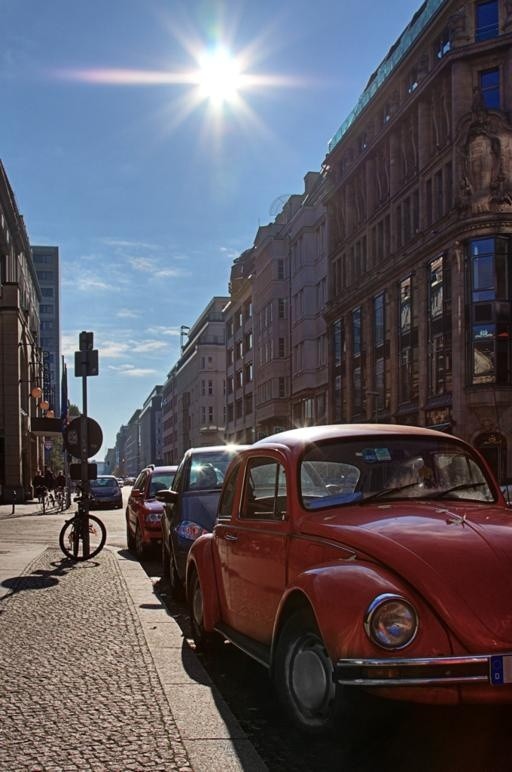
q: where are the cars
[88,474,137,510]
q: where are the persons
[33,467,65,507]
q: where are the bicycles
[58,491,106,559]
